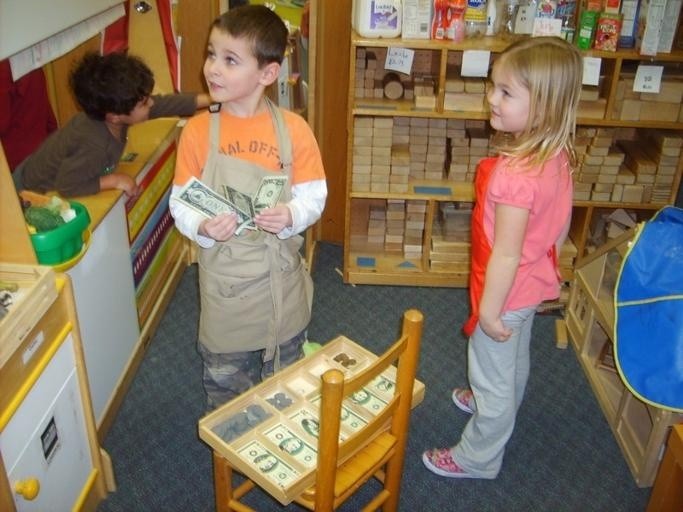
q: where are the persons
[11,52,219,210]
[167,3,329,416]
[420,35,585,481]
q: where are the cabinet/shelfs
[343,27,683,288]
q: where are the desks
[0,118,196,445]
[0,264,117,512]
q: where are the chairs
[212,308,422,512]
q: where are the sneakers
[421,447,498,478]
[452,387,478,415]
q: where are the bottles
[563,13,576,44]
[432,0,518,43]
